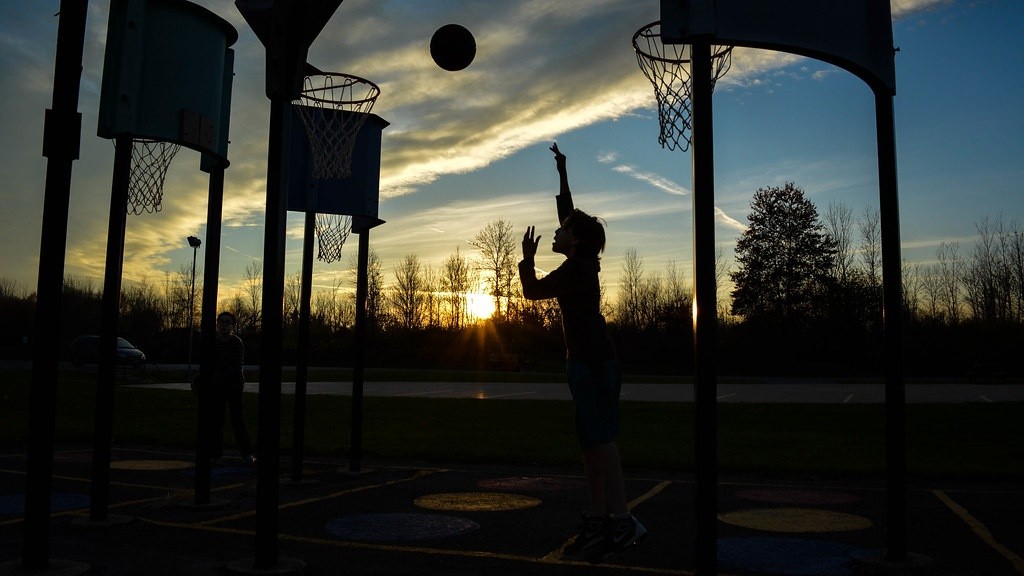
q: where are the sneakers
[594,514,650,564]
[565,515,611,562]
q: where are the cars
[71,335,147,373]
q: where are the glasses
[218,320,234,326]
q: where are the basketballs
[429,24,477,72]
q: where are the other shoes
[212,458,224,469]
[241,455,257,467]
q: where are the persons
[211,312,258,468]
[519,141,646,563]
